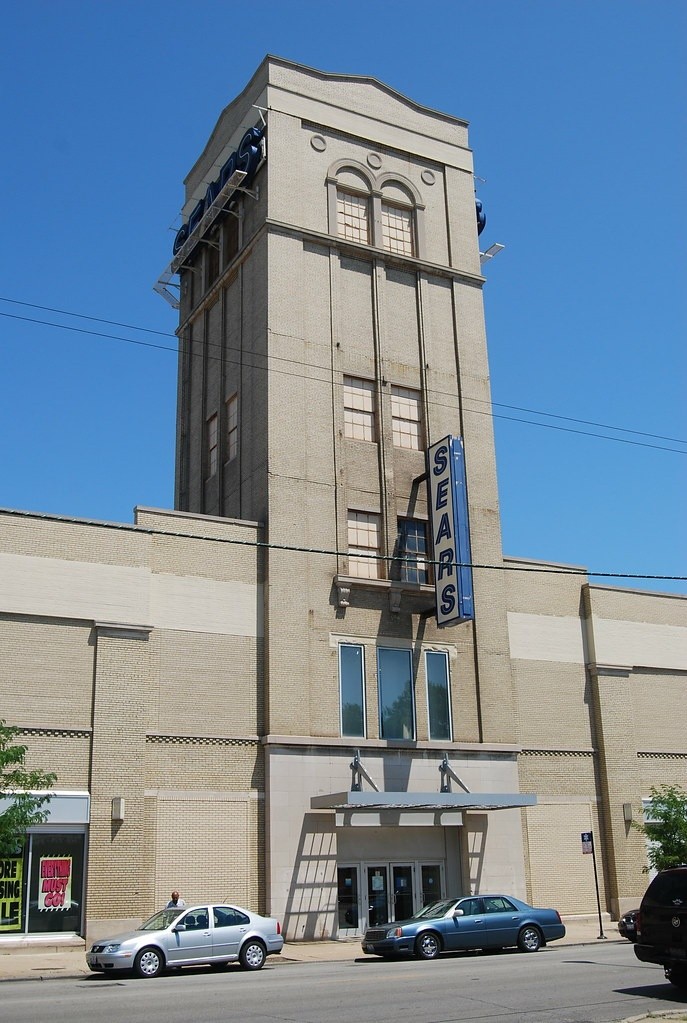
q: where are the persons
[166,891,186,970]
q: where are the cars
[86,902,284,979]
[634,865,687,990]
[361,893,566,961]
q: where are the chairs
[197,915,207,929]
[185,916,196,929]
[232,916,242,925]
[221,915,232,926]
